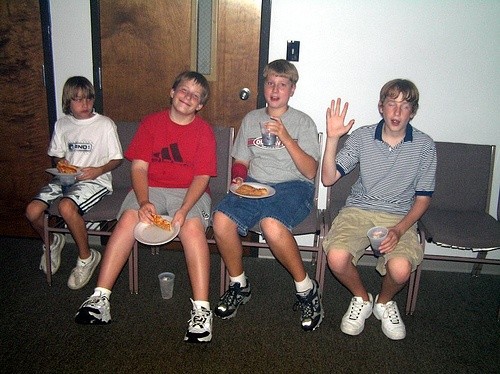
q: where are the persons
[214,59,323,332]
[26,76,126,291]
[74,72,218,344]
[321,79,438,339]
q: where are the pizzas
[236,184,268,195]
[56,162,77,174]
[150,212,171,231]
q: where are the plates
[46,168,82,177]
[134,214,180,246]
[230,182,277,199]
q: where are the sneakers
[185,297,213,343]
[213,276,252,320]
[371,294,407,341]
[340,292,374,336]
[73,290,112,326]
[66,248,102,291]
[292,278,325,333]
[39,232,66,276]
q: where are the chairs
[225,132,323,284]
[43,121,154,295]
[411,142,500,313]
[133,124,235,296]
[319,134,416,315]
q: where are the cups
[259,120,276,146]
[158,272,175,300]
[59,174,76,196]
[367,226,390,257]
